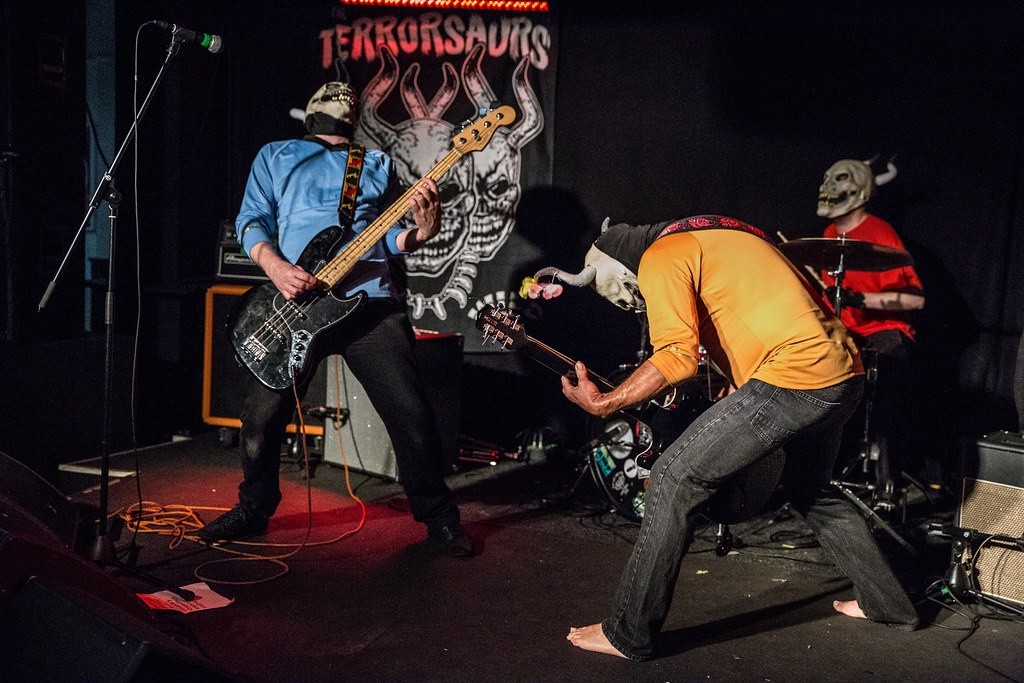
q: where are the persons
[815,158,925,379]
[560,214,922,662]
[195,80,474,559]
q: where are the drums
[857,346,880,415]
[585,362,719,523]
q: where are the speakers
[322,355,404,484]
[0,453,235,683]
[202,284,326,436]
[948,429,1024,611]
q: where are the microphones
[310,407,350,417]
[153,20,222,53]
[584,424,629,451]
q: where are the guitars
[475,302,718,472]
[225,103,516,390]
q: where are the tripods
[910,525,1023,620]
[35,34,196,603]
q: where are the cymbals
[780,237,913,272]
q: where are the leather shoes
[427,522,474,556]
[198,503,269,541]
